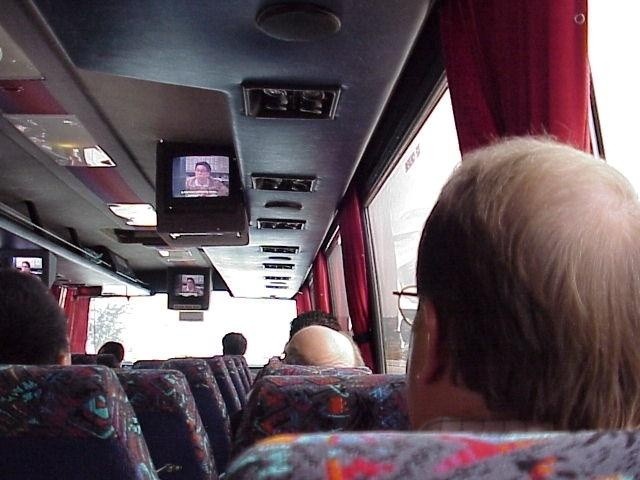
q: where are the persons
[0,267,73,366]
[222,332,247,355]
[290,311,365,365]
[99,342,124,362]
[404,136,640,432]
[285,325,355,367]
[21,261,35,274]
[185,162,229,196]
[183,278,202,294]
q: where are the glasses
[391,283,422,329]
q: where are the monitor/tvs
[10,251,57,289]
[168,268,210,310]
[156,142,245,233]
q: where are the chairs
[0,354,640,480]
